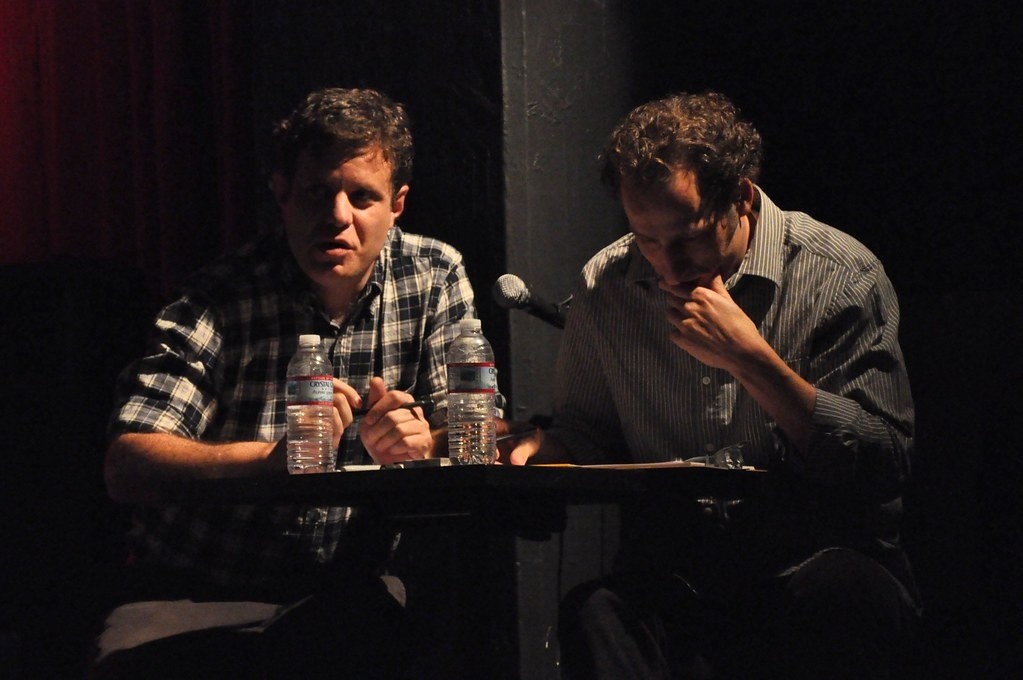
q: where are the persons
[487,92,932,680]
[93,91,504,680]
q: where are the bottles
[448,318,498,466]
[285,335,334,475]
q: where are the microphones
[492,273,566,331]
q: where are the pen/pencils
[345,398,437,417]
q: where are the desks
[257,459,793,680]
